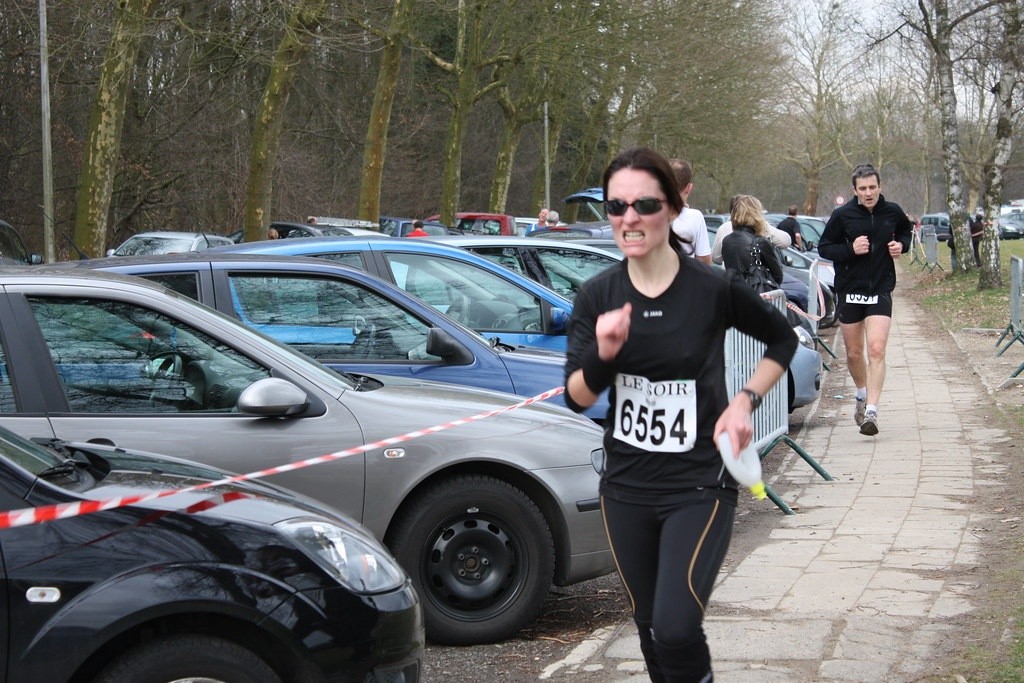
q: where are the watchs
[741,388,762,406]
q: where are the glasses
[603,198,669,217]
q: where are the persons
[948,214,984,269]
[778,205,803,251]
[306,217,317,223]
[714,194,791,292]
[818,164,914,436]
[408,221,430,237]
[670,160,711,264]
[526,208,559,235]
[563,147,793,683]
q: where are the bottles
[719,431,767,501]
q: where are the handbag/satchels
[748,238,779,294]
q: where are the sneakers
[861,409,878,436]
[854,395,868,426]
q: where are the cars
[920,200,1024,241]
[0,263,615,682]
[104,188,832,467]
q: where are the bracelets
[583,353,613,395]
